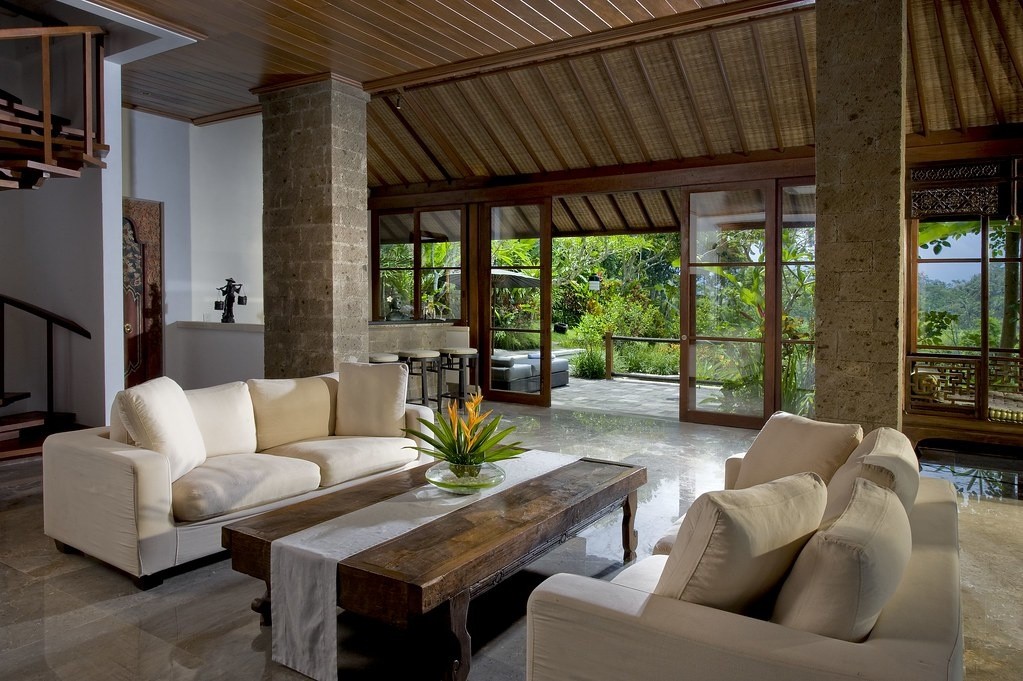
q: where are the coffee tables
[220,444,647,681]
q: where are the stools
[369,353,399,364]
[439,347,479,416]
[396,349,442,415]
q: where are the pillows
[335,361,409,438]
[115,376,207,484]
[654,471,827,621]
[734,410,863,490]
[816,427,921,528]
[770,476,912,643]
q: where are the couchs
[42,404,441,593]
[526,453,963,680]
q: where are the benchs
[491,357,569,393]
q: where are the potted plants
[398,391,525,496]
